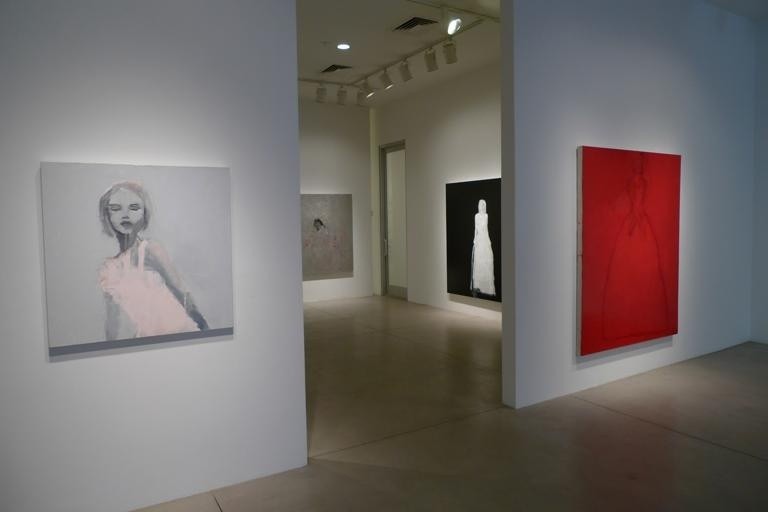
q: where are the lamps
[352,34,460,99]
[298,77,370,107]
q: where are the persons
[469,199,497,297]
[94,180,210,339]
